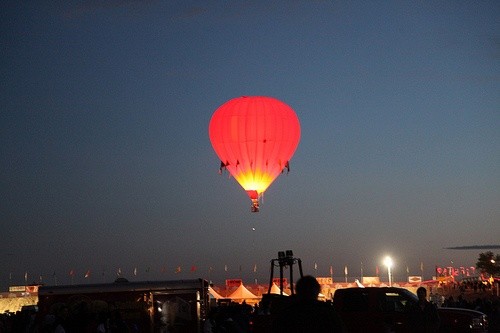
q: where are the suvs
[333,287,490,333]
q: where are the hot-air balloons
[208,96,301,213]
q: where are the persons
[417,287,434,310]
[441,295,500,333]
[0,276,345,333]
